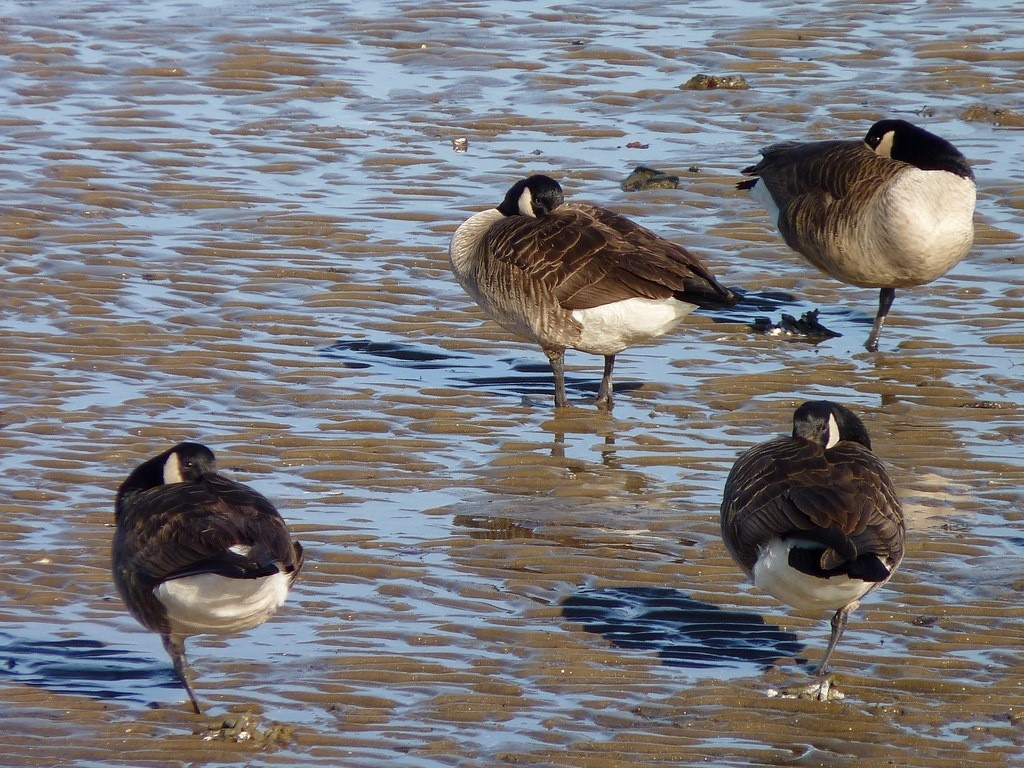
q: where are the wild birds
[110,441,305,716]
[734,118,977,347]
[720,400,907,677]
[448,173,746,409]
